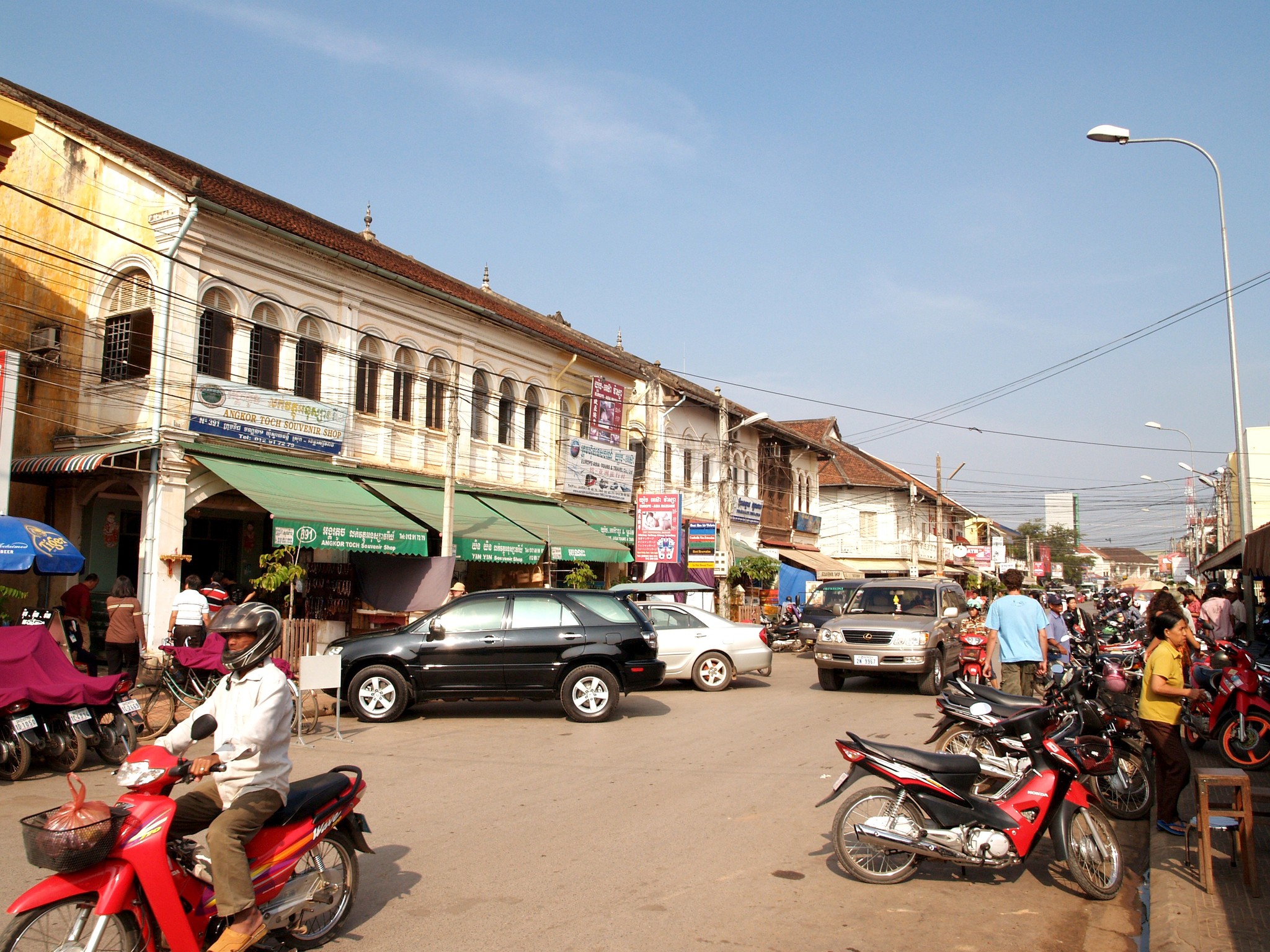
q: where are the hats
[1224,587,1238,595]
[450,582,465,593]
[1048,595,1063,605]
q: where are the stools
[1185,816,1238,870]
[1194,767,1259,898]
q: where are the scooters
[0,677,148,782]
[777,596,800,626]
[947,622,988,684]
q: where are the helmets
[1118,593,1128,599]
[206,601,283,671]
[1121,596,1132,607]
[1074,734,1114,772]
[1102,664,1126,693]
[1205,582,1222,597]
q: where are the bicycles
[128,635,319,742]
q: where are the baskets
[1100,691,1136,713]
[1099,704,1143,737]
[137,655,163,686]
[1057,741,1120,776]
[19,802,131,871]
[1102,675,1129,695]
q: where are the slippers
[1156,818,1188,836]
[207,922,268,952]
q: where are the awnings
[9,440,163,473]
[1196,521,1270,578]
[177,439,661,564]
[766,537,865,581]
[833,557,1039,587]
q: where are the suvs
[730,609,772,677]
[813,579,971,695]
[322,588,667,724]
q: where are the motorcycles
[923,597,1270,820]
[813,703,1126,900]
[759,611,809,653]
[1,712,376,952]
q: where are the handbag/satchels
[38,772,111,856]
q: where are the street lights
[1140,508,1169,557]
[1140,475,1177,553]
[717,398,770,619]
[1145,421,1202,588]
[1085,127,1261,642]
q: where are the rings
[200,767,205,770]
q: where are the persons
[59,574,100,657]
[1028,592,1046,615]
[105,575,148,696]
[953,598,1000,689]
[168,575,212,690]
[1137,576,1256,838]
[994,591,1005,600]
[149,601,300,952]
[197,569,256,640]
[965,591,984,619]
[441,582,469,609]
[1061,597,1094,647]
[1042,595,1074,695]
[983,568,1050,702]
[1092,590,1144,642]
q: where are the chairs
[868,595,894,612]
[828,594,843,607]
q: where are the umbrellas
[1136,580,1169,591]
[0,510,86,575]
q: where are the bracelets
[168,631,172,632]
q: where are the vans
[798,579,884,647]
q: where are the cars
[1032,583,1155,608]
[603,601,774,692]
